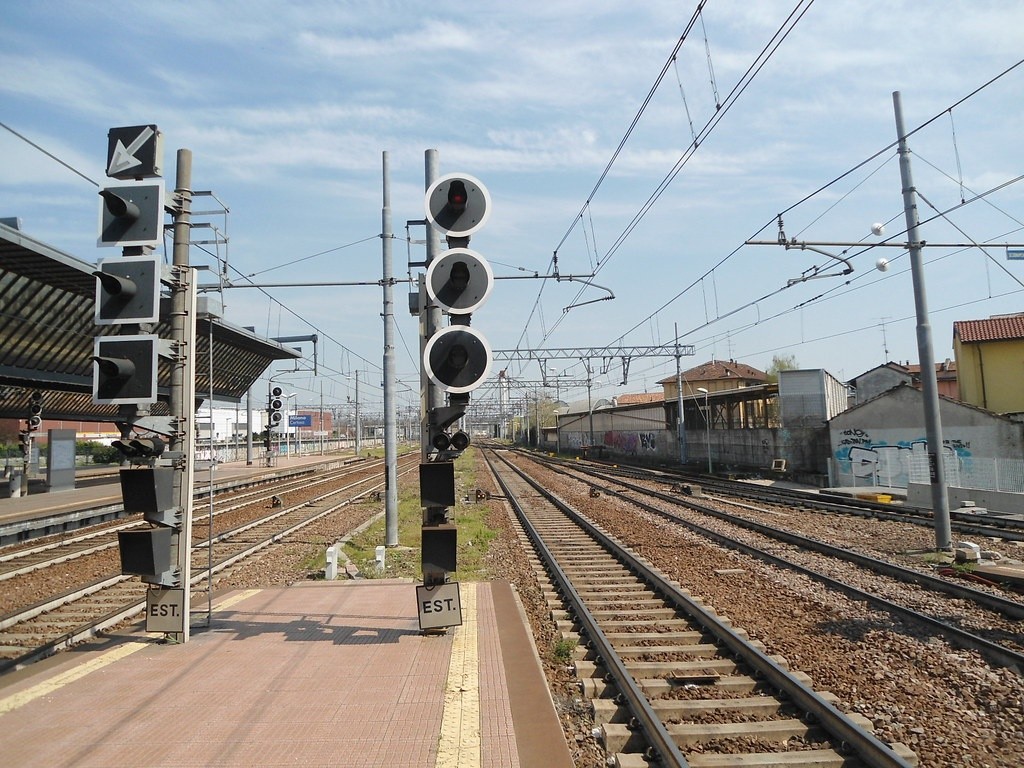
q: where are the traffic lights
[91,256,161,328]
[423,324,493,394]
[87,333,160,406]
[420,520,458,575]
[30,416,42,427]
[272,387,282,396]
[416,460,458,509]
[110,438,164,458]
[117,527,172,576]
[31,404,43,416]
[95,179,167,247]
[271,412,283,423]
[271,399,283,410]
[118,467,174,514]
[31,391,42,402]
[423,172,492,238]
[432,428,470,452]
[425,247,495,316]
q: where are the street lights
[280,393,298,460]
[695,387,713,474]
[345,375,360,456]
